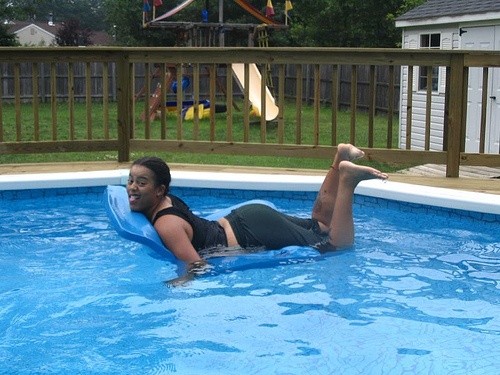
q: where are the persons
[127,141,389,284]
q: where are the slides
[231,62,279,122]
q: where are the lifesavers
[105,185,321,256]
